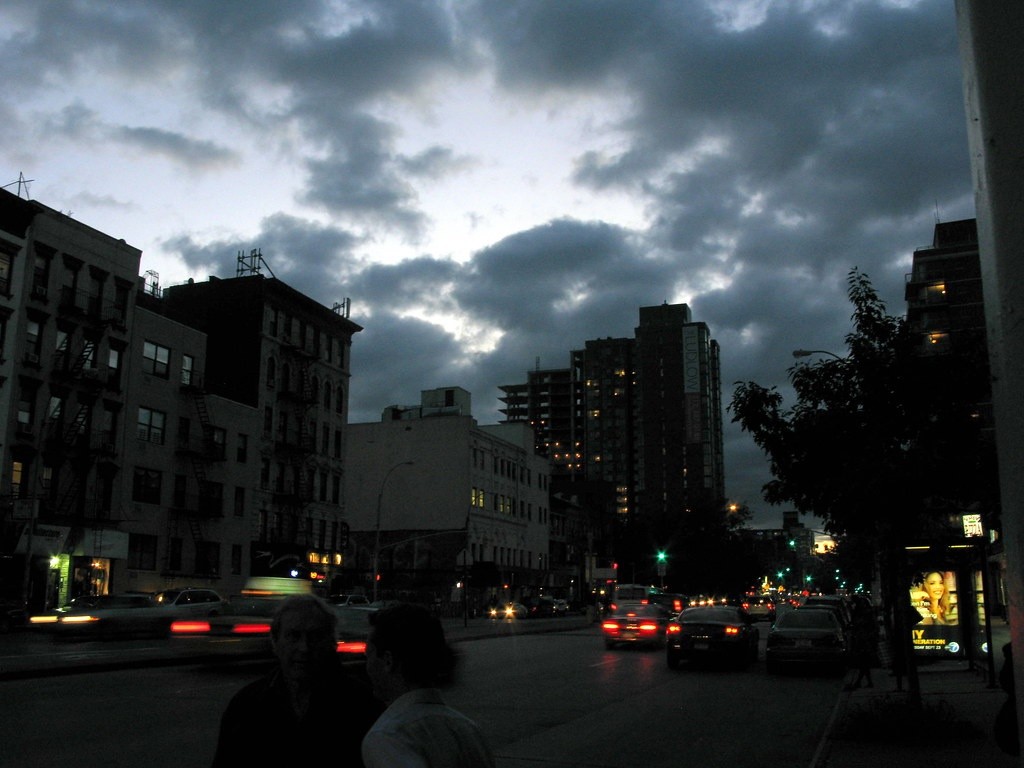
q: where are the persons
[915,572,948,625]
[893,595,923,691]
[211,593,495,768]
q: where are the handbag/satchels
[877,639,896,665]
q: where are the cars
[616,579,875,637]
[664,606,760,668]
[766,608,847,668]
[487,597,570,621]
[29,573,330,665]
[330,593,368,609]
[599,603,670,649]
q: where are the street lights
[372,460,416,602]
[463,490,495,627]
